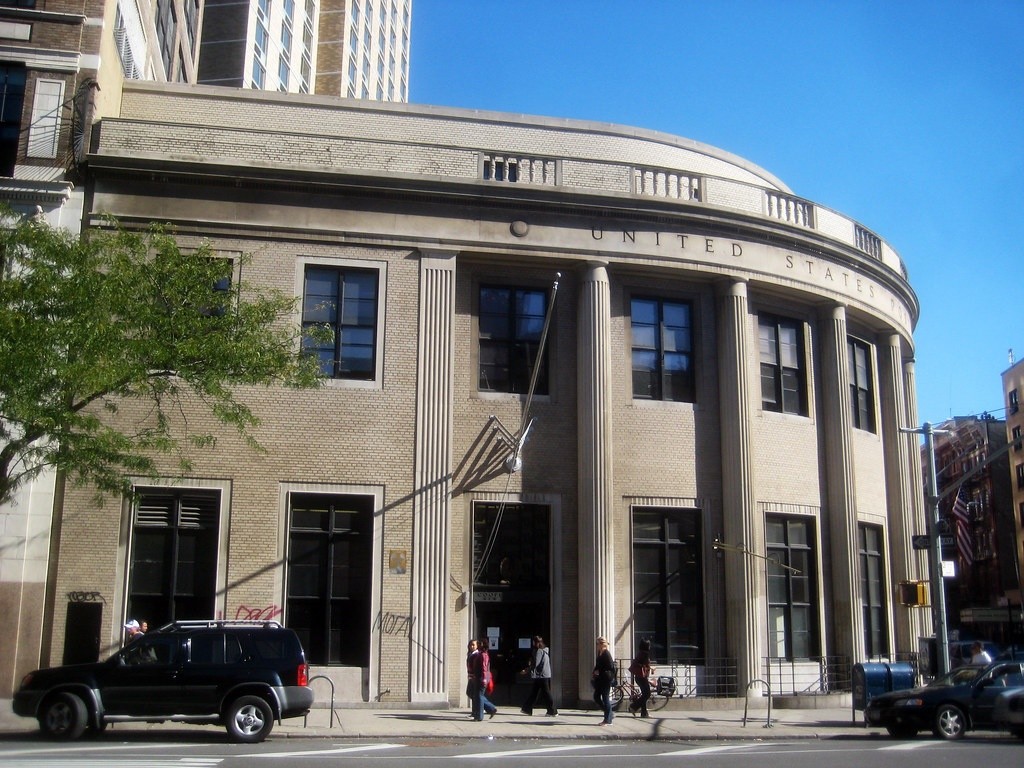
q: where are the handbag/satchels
[627,653,650,678]
[531,650,545,675]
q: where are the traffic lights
[898,582,927,608]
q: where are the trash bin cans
[852,662,916,730]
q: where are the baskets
[657,676,675,696]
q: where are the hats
[970,641,984,648]
[124,620,139,628]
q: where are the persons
[520,635,559,717]
[466,637,497,722]
[593,637,615,726]
[629,639,656,718]
[124,620,147,645]
[969,641,991,664]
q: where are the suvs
[13,619,314,743]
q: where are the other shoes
[630,707,636,717]
[490,708,497,719]
[598,711,616,727]
[641,714,654,718]
[545,709,558,717]
[521,708,532,715]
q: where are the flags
[953,482,974,567]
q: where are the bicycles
[602,667,669,711]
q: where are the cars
[862,640,1024,740]
[655,645,699,664]
